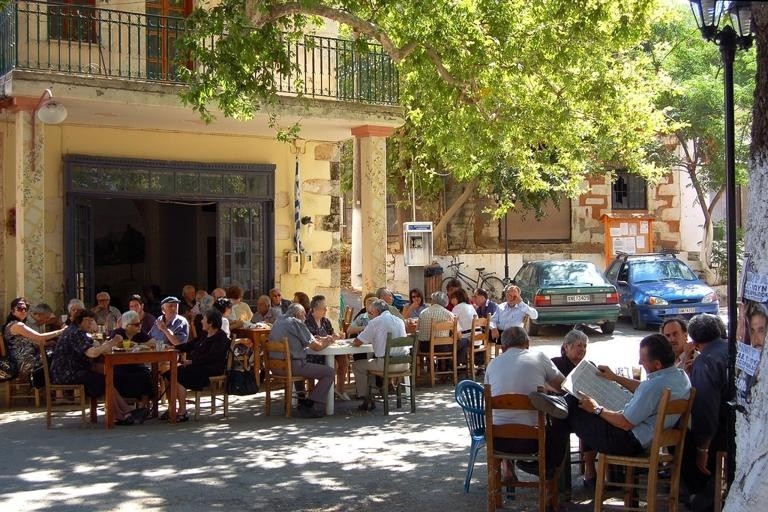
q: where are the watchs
[696,446,709,453]
[594,406,603,416]
[164,328,170,333]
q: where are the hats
[160,296,182,306]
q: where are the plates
[112,342,138,352]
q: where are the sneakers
[335,390,350,401]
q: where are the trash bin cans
[425,260,444,303]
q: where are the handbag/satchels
[0,329,16,381]
[224,344,258,395]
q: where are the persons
[666,314,729,512]
[194,295,214,338]
[223,285,253,323]
[249,295,282,382]
[444,279,469,312]
[211,288,226,305]
[178,285,193,315]
[547,330,599,489]
[108,310,156,408]
[436,288,483,384]
[516,333,693,480]
[657,319,696,478]
[417,291,487,365]
[161,307,231,422]
[291,292,311,317]
[268,303,333,416]
[353,299,411,410]
[65,299,85,328]
[50,308,137,422]
[484,326,569,482]
[147,296,189,377]
[303,295,352,401]
[376,287,415,326]
[489,285,539,344]
[347,297,379,338]
[474,288,497,375]
[3,297,80,406]
[747,303,768,348]
[269,288,291,314]
[91,292,121,326]
[129,294,156,336]
[188,291,208,322]
[350,293,383,400]
[212,298,233,341]
[403,288,437,372]
[24,303,56,347]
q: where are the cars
[502,258,621,336]
[601,251,719,330]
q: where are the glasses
[270,294,283,299]
[127,322,142,327]
[17,307,29,311]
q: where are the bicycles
[439,261,506,305]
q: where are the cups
[155,340,164,352]
[122,339,131,353]
[631,367,641,381]
[162,344,169,351]
[97,323,108,334]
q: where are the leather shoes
[359,401,374,410]
[514,460,554,482]
[526,393,569,420]
[298,405,324,419]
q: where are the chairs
[454,380,518,501]
[192,315,249,374]
[194,334,237,422]
[415,316,459,389]
[457,314,490,382]
[39,343,86,430]
[592,386,698,511]
[684,443,733,512]
[259,335,315,418]
[367,333,420,416]
[482,383,558,511]
[495,315,530,360]
[0,334,40,408]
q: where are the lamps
[31,88,68,128]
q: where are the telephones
[409,236,423,250]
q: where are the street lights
[689,0,757,505]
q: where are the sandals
[176,411,190,421]
[114,418,128,426]
[159,410,169,420]
[126,409,146,423]
